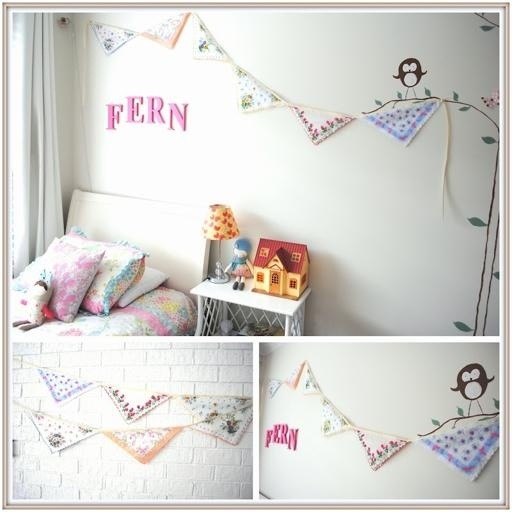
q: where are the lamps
[203,203,240,284]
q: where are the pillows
[11,225,171,333]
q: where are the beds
[9,186,209,338]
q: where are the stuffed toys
[221,238,255,291]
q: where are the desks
[189,274,312,338]
